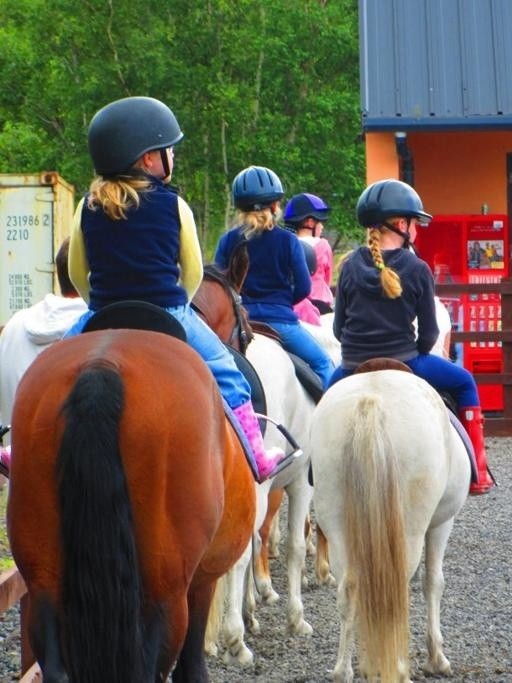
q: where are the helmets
[358,178,432,228]
[285,193,330,224]
[88,95,185,175]
[232,164,284,208]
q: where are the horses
[5,239,473,683]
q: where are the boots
[231,400,286,480]
[0,445,11,467]
[459,406,493,494]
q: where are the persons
[1,237,90,450]
[213,164,336,387]
[284,192,333,314]
[470,242,498,263]
[1,95,286,478]
[323,178,494,493]
[412,295,451,360]
